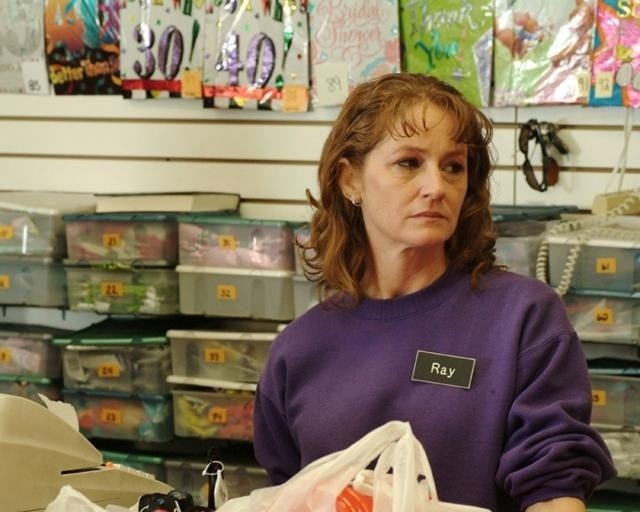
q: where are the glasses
[519,119,558,192]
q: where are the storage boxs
[488,193,638,487]
[1,191,328,508]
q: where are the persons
[254,73,616,512]
